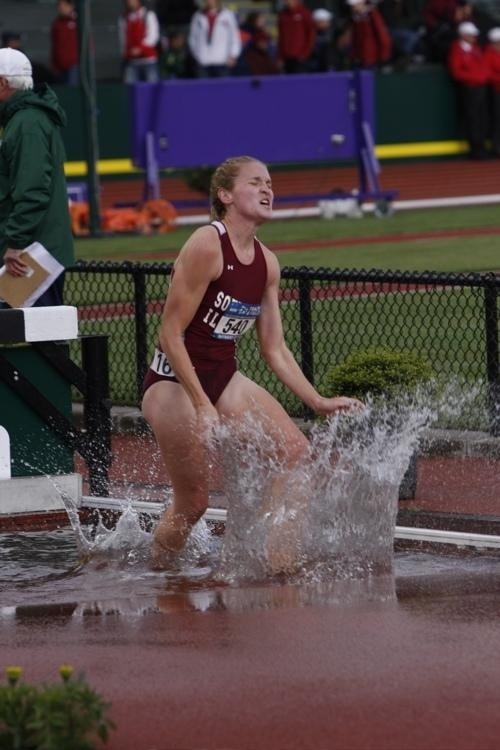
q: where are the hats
[0,46,32,77]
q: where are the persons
[186,0,241,79]
[117,1,159,85]
[132,152,369,570]
[421,1,500,163]
[0,43,79,477]
[50,0,79,86]
[160,31,190,79]
[235,1,393,72]
